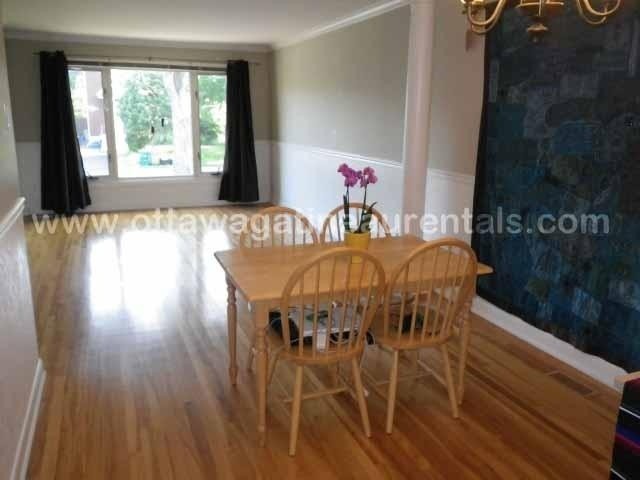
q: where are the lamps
[458,1,622,52]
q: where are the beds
[213,233,493,433]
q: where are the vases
[344,232,371,264]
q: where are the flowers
[337,162,378,232]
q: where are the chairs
[267,247,384,457]
[360,238,478,434]
[239,206,320,371]
[320,204,416,308]
